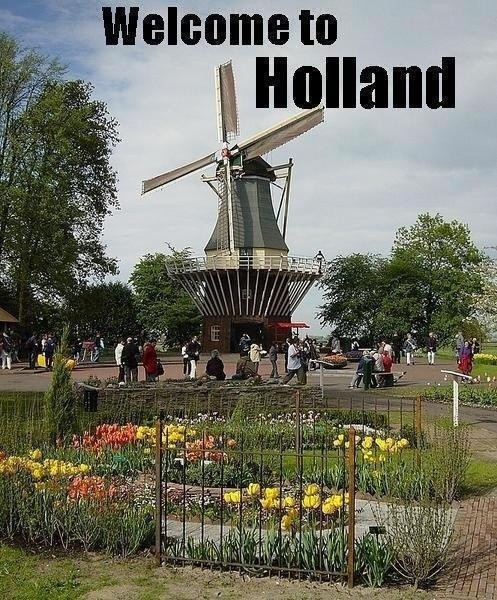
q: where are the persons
[114,336,159,382]
[181,331,417,390]
[425,332,436,365]
[456,330,479,384]
[0,328,102,372]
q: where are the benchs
[358,372,393,388]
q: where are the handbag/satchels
[232,370,245,380]
[158,361,164,375]
